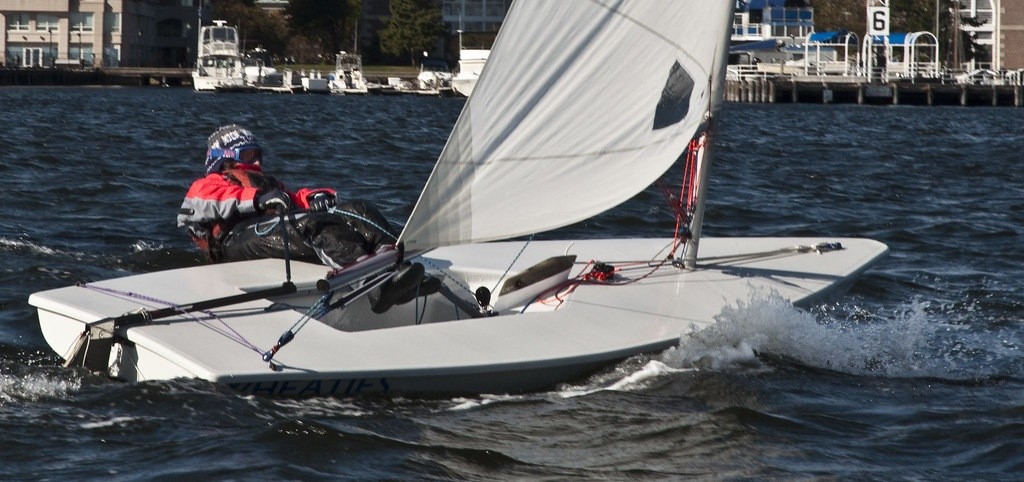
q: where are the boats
[189,20,488,99]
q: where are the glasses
[207,146,262,164]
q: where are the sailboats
[28,0,893,408]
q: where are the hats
[206,124,257,175]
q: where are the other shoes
[364,262,425,313]
[392,276,440,305]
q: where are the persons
[177,123,440,314]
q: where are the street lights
[77,32,82,68]
[48,29,53,67]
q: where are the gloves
[258,191,292,212]
[310,191,337,212]
